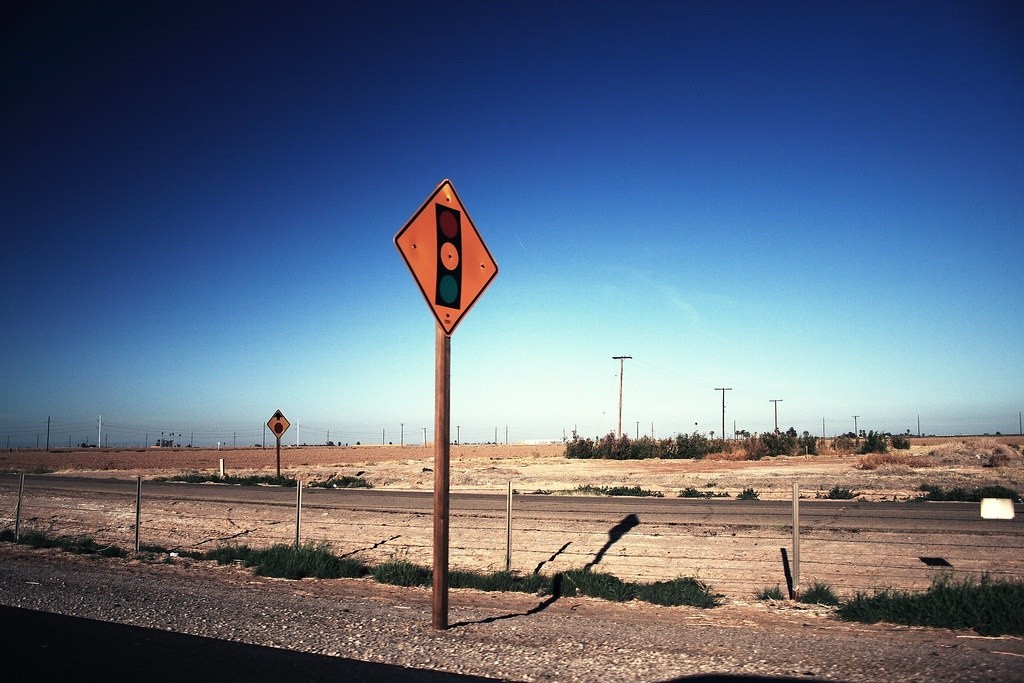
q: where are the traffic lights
[434,201,462,315]
[274,423,283,434]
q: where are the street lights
[422,427,427,445]
[637,422,640,441]
[769,399,784,432]
[715,387,733,441]
[851,414,860,438]
[612,356,631,440]
[457,426,461,447]
